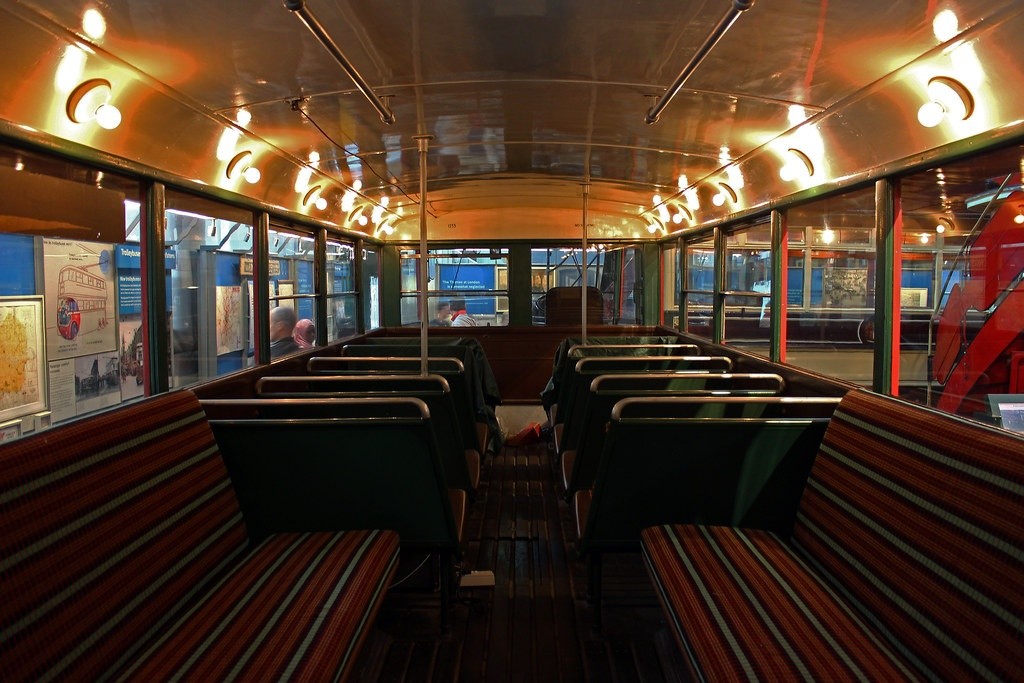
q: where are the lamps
[936,216,955,234]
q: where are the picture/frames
[0,295,49,422]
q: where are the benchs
[198,337,846,646]
[0,384,403,683]
[640,386,1024,683]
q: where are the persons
[269,306,316,358]
[430,300,476,327]
